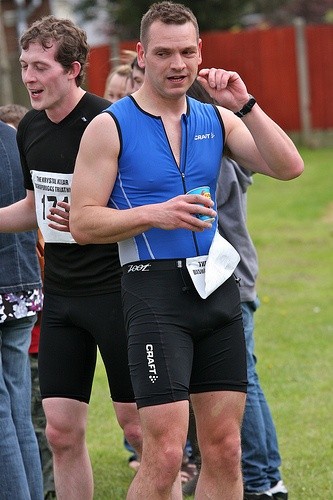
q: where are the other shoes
[179,461,203,497]
[127,459,144,474]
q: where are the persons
[0,2,304,500]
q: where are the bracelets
[234,94,256,118]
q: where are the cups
[186,186,215,224]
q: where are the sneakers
[243,488,274,500]
[270,479,289,500]
